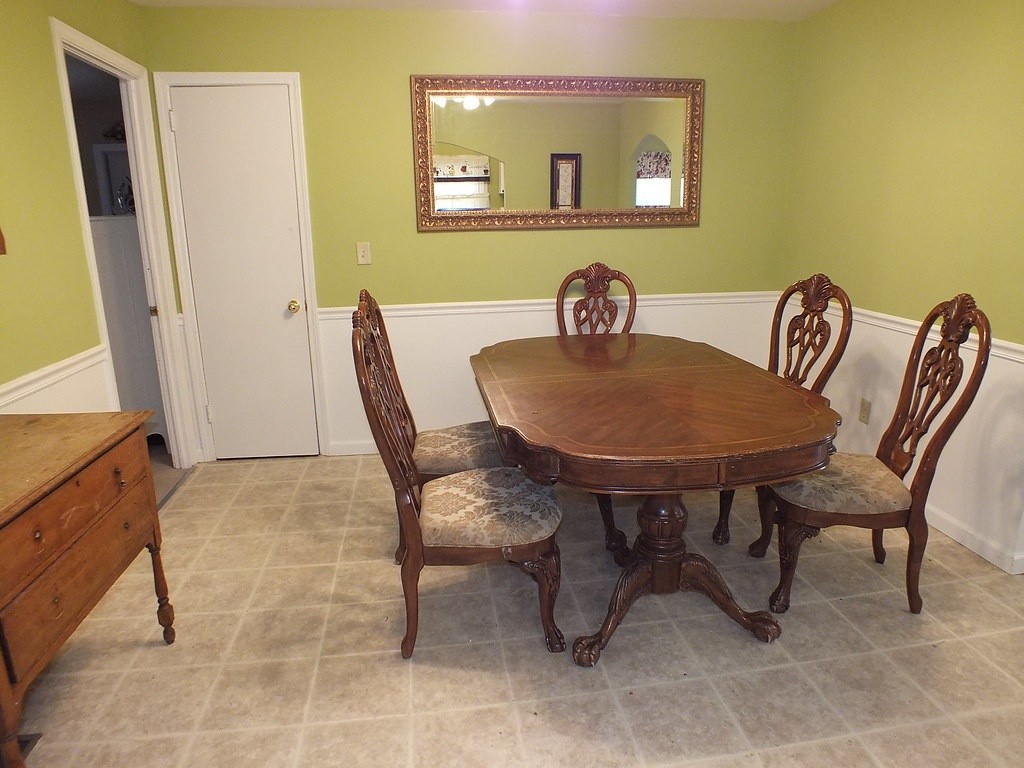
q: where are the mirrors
[407,72,702,232]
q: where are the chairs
[744,294,996,616]
[353,289,517,565]
[351,310,568,659]
[553,262,639,338]
[708,272,854,543]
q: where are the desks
[469,332,844,667]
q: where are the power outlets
[857,397,871,424]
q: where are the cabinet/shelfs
[1,409,181,768]
